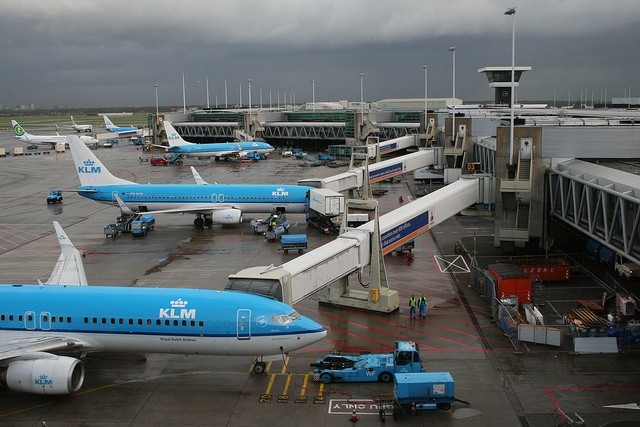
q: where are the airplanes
[70,116,93,132]
[10,119,99,150]
[0,220,328,394]
[150,120,275,162]
[103,116,139,132]
[60,134,318,228]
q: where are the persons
[416,294,428,319]
[408,249,413,263]
[408,294,418,320]
[398,194,403,206]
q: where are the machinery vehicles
[310,340,426,385]
[305,188,346,235]
[131,135,145,146]
[131,215,155,237]
[46,189,63,204]
[103,138,119,148]
[282,148,307,159]
[151,157,170,166]
